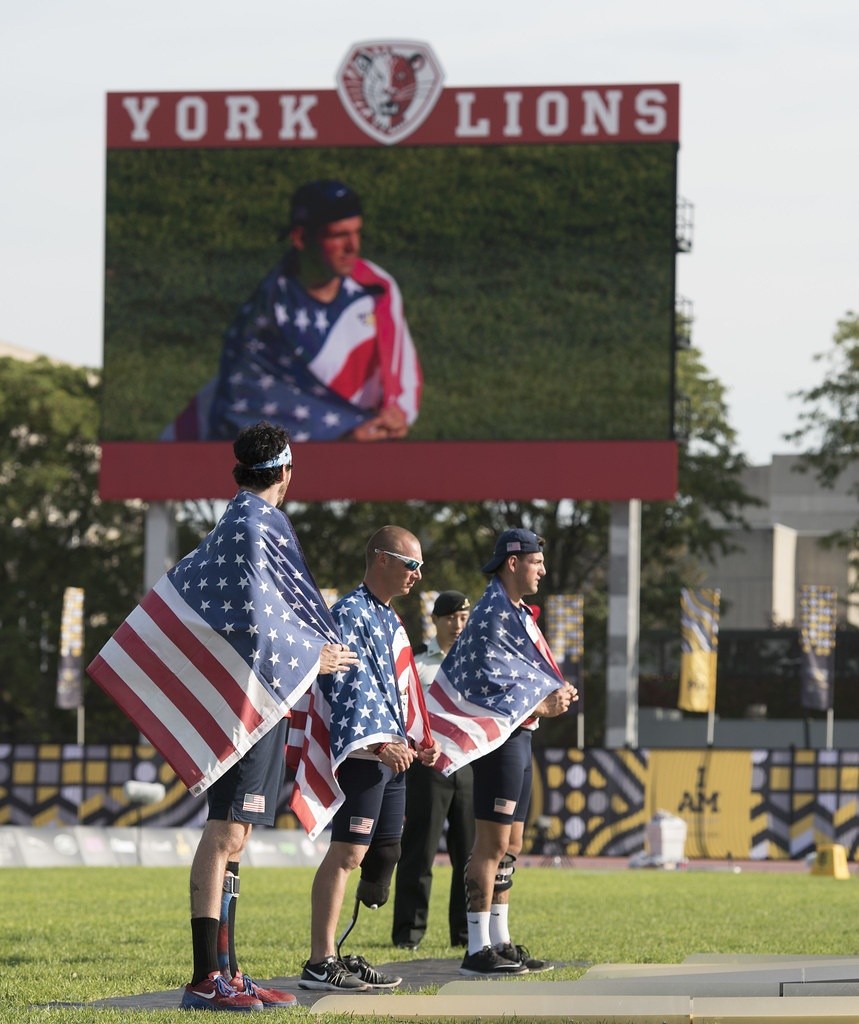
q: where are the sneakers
[182,970,298,1012]
[459,943,555,976]
[299,959,372,992]
[341,955,402,989]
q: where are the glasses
[375,548,424,570]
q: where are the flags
[317,589,339,609]
[543,593,584,688]
[421,592,441,646]
[798,585,836,710]
[676,588,720,712]
[55,585,85,709]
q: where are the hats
[289,179,362,228]
[433,591,470,617]
[482,529,544,573]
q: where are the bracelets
[374,743,388,755]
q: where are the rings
[340,643,344,651]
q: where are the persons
[425,529,579,976]
[158,179,420,443]
[86,420,351,1011]
[391,590,476,950]
[286,525,441,992]
